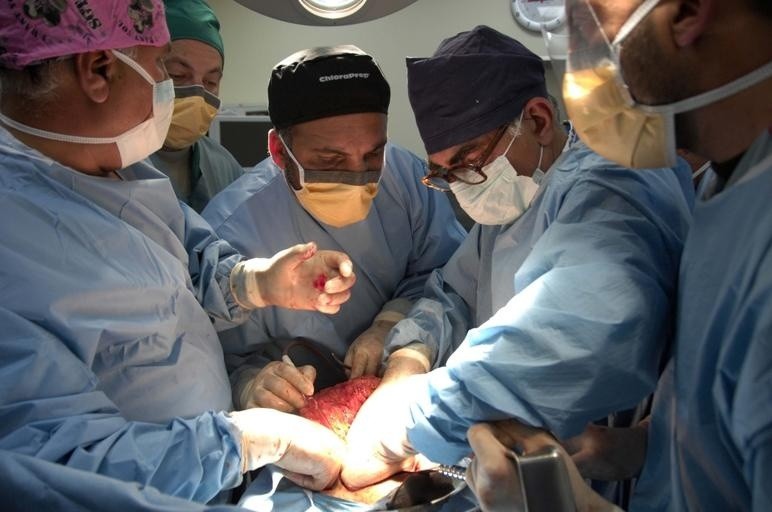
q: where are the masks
[449,110,545,226]
[280,135,387,227]
[162,83,222,153]
[561,2,678,169]
[112,49,176,171]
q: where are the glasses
[419,113,510,194]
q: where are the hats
[402,23,551,158]
[163,0,231,71]
[0,0,171,71]
[266,42,392,130]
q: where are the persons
[461,0,768,512]
[199,41,472,411]
[0,0,360,512]
[142,0,247,218]
[339,22,694,494]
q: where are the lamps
[237,1,423,27]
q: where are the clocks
[509,0,566,33]
